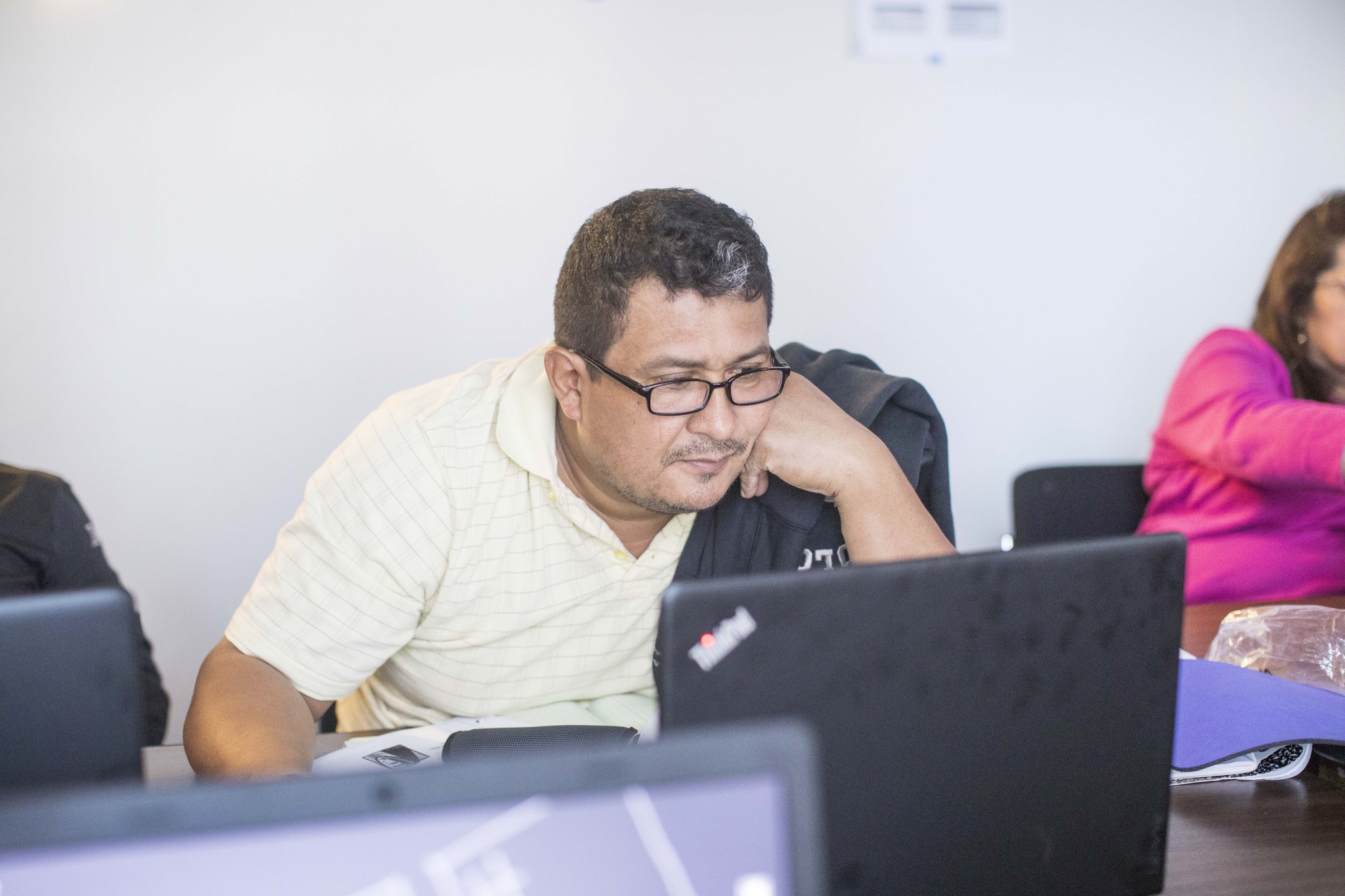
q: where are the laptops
[0,585,143,811]
[659,534,1188,896]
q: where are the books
[1169,646,1314,785]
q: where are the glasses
[567,345,791,417]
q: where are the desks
[135,728,1345,896]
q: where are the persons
[1130,189,1345,605]
[1,461,170,745]
[184,188,957,779]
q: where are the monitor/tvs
[0,719,833,896]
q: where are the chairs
[0,587,140,796]
[1013,464,1148,546]
